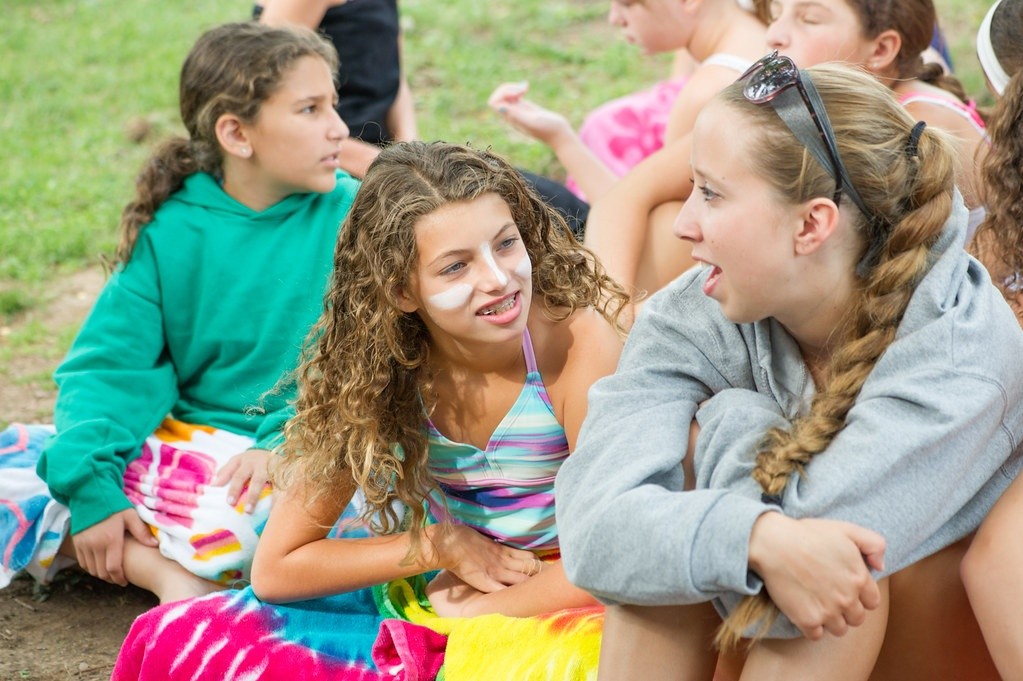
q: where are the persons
[0,16,370,612]
[249,0,417,181]
[972,1,1023,105]
[547,50,1023,681]
[105,136,630,680]
[484,0,771,206]
[583,0,1023,331]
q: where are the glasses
[738,50,842,206]
